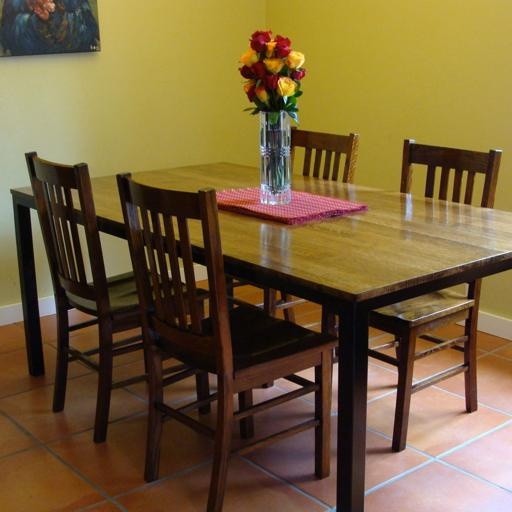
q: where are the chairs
[229,125,360,388]
[25,150,213,445]
[321,138,502,453]
[113,166,339,512]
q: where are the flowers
[239,25,307,192]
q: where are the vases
[259,110,289,205]
